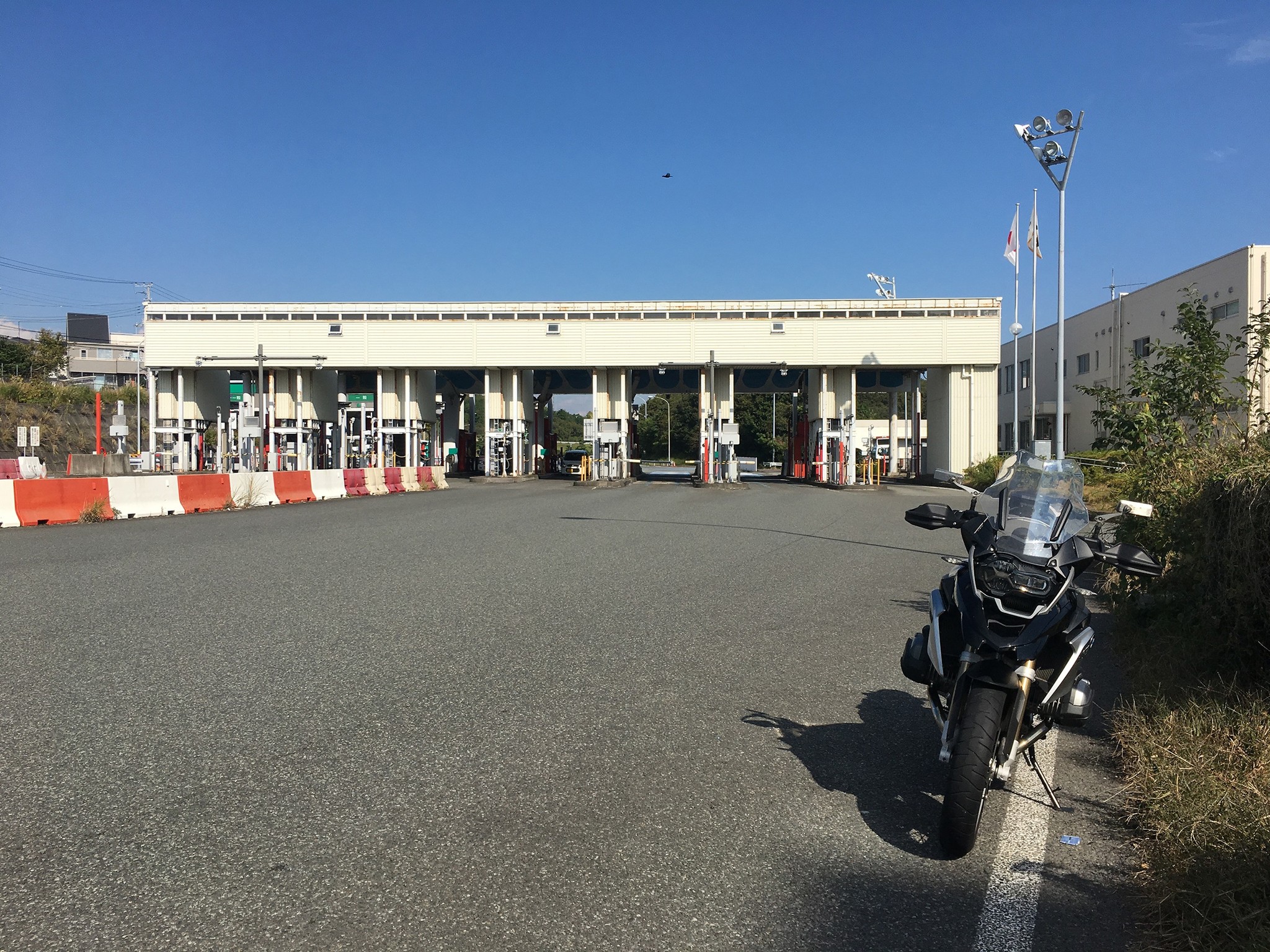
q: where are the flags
[1028,208,1042,259]
[1003,211,1020,265]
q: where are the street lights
[648,396,670,461]
[1013,109,1084,474]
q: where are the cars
[561,450,593,477]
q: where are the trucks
[874,438,889,470]
[477,441,485,472]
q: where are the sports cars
[900,452,1161,854]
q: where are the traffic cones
[40,462,49,479]
